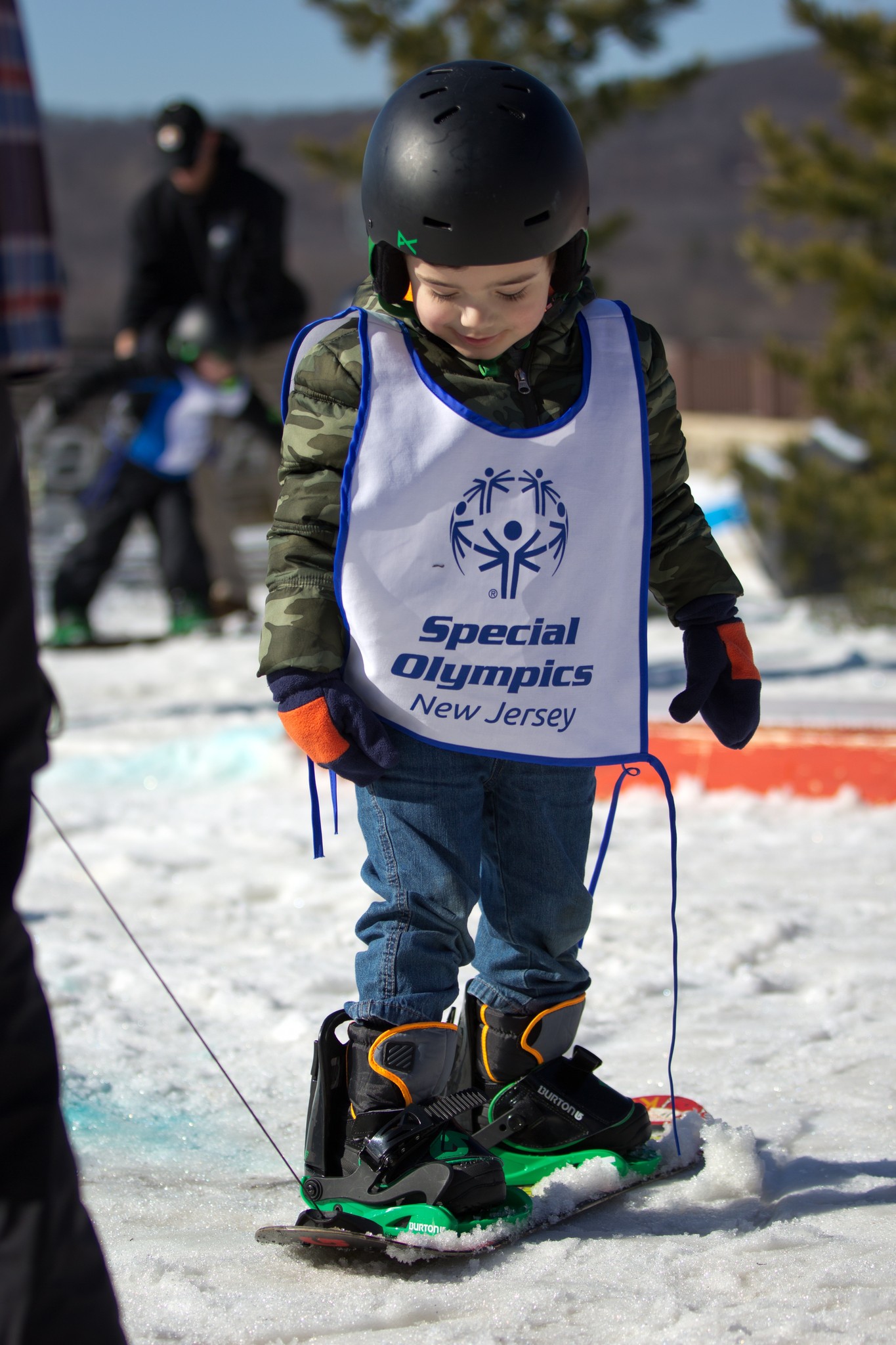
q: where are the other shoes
[175,606,220,635]
[53,605,92,647]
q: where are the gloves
[268,664,399,786]
[668,615,762,751]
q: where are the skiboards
[37,621,254,656]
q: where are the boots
[452,977,652,1156]
[345,1020,507,1216]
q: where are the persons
[50,299,284,644]
[113,101,309,617]
[298,54,765,1233]
[0,399,126,1345]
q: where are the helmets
[363,61,590,303]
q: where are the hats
[152,102,204,169]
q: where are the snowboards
[254,1095,718,1263]
